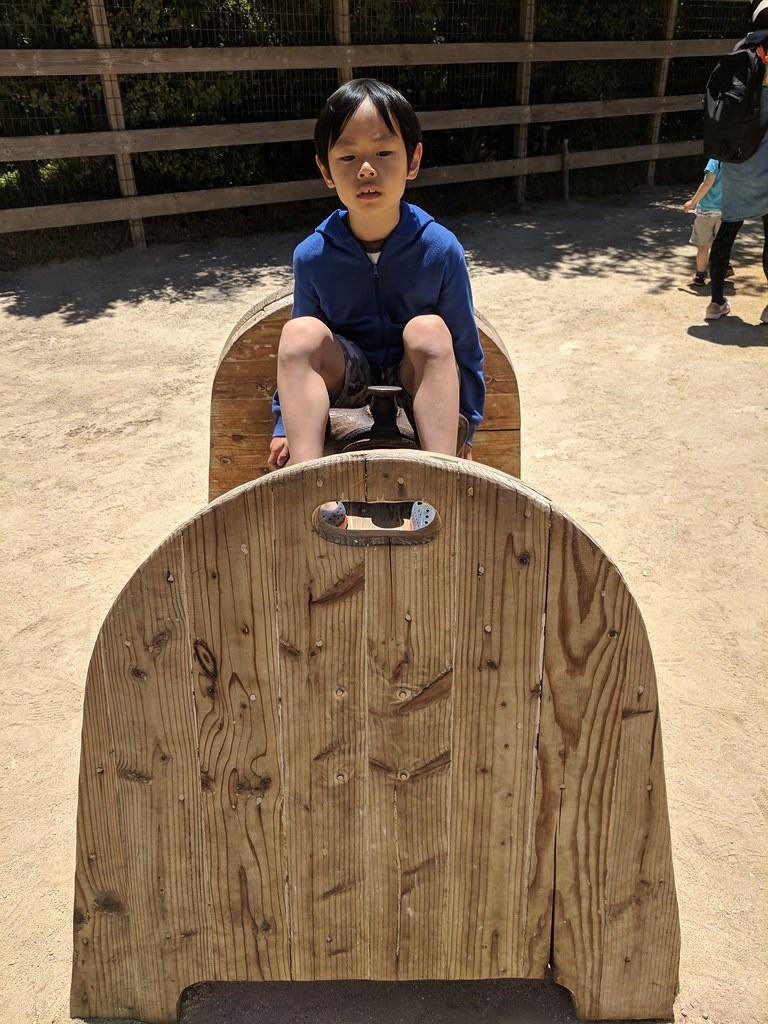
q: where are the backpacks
[702,30,768,164]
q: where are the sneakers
[725,266,734,277]
[706,297,730,319]
[760,305,768,322]
[695,271,707,285]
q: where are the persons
[680,0,768,322]
[266,79,484,470]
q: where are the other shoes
[319,501,347,529]
[410,501,436,531]
